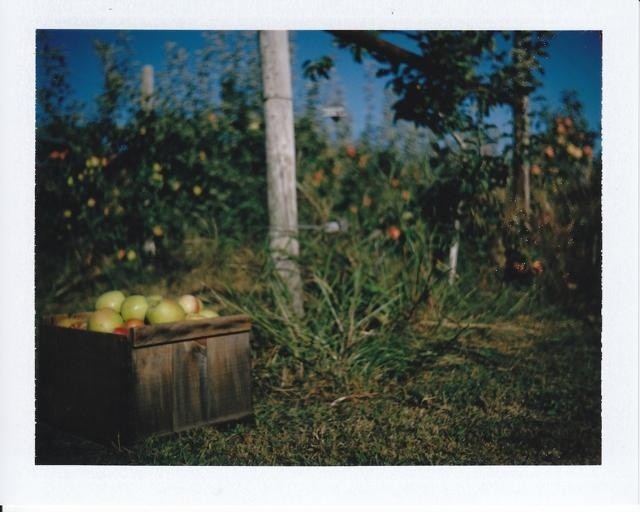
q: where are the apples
[57,291,219,335]
